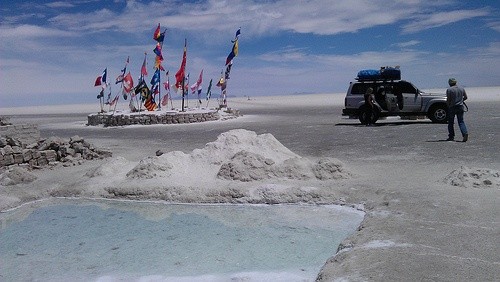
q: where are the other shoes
[447,136,454,140]
[463,133,468,142]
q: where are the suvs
[342,65,448,126]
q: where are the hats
[448,78,458,83]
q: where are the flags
[94,23,240,111]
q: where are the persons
[364,87,375,126]
[446,77,469,142]
[372,86,384,126]
